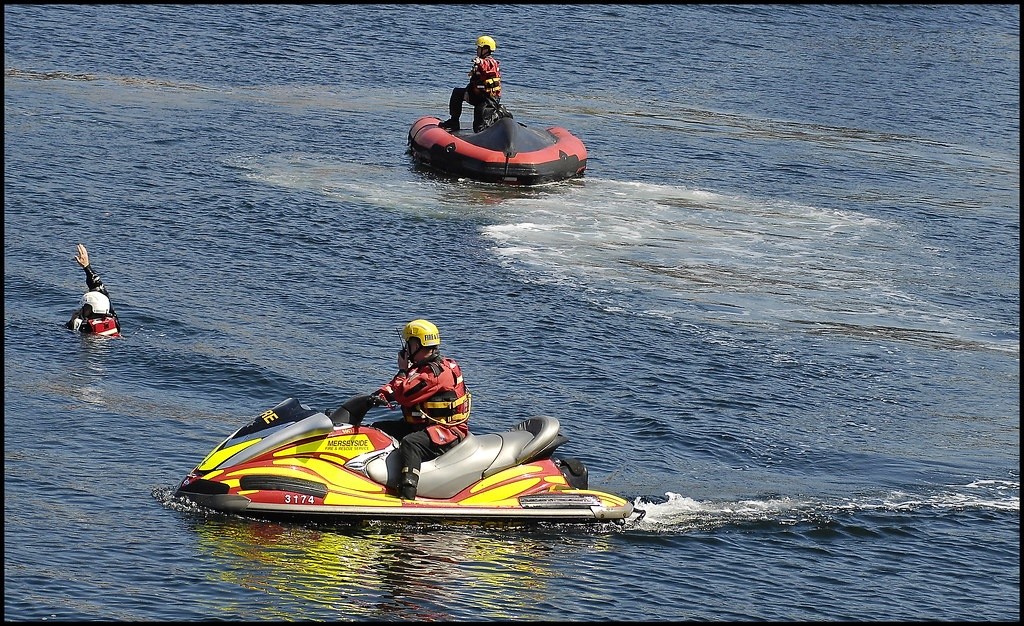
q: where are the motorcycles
[171,394,634,521]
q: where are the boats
[405,115,588,185]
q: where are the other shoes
[498,103,514,120]
[389,484,417,501]
[438,118,460,131]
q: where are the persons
[438,36,514,133]
[372,319,471,500]
[65,244,121,339]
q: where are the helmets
[476,35,496,51]
[79,291,110,314]
[402,319,441,347]
